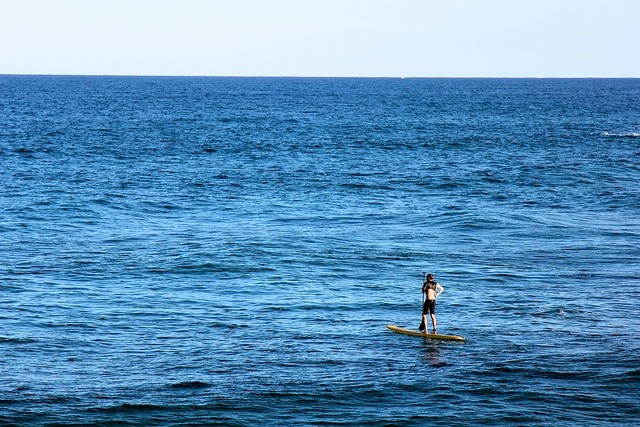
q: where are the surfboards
[386,324,465,341]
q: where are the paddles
[418,272,425,331]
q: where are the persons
[422,274,444,335]
